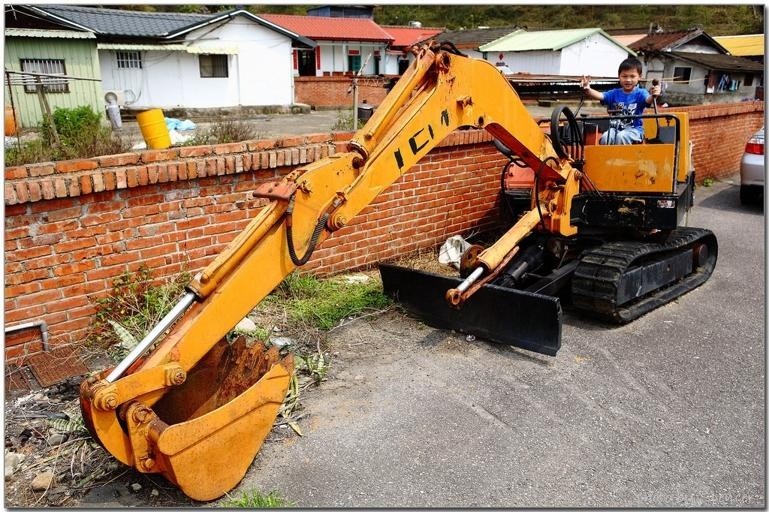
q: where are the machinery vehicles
[75,39,718,502]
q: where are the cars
[738,121,765,207]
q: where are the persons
[579,57,662,145]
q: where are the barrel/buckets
[358,104,373,124]
[105,104,122,129]
[4,107,18,136]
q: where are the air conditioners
[105,90,125,106]
